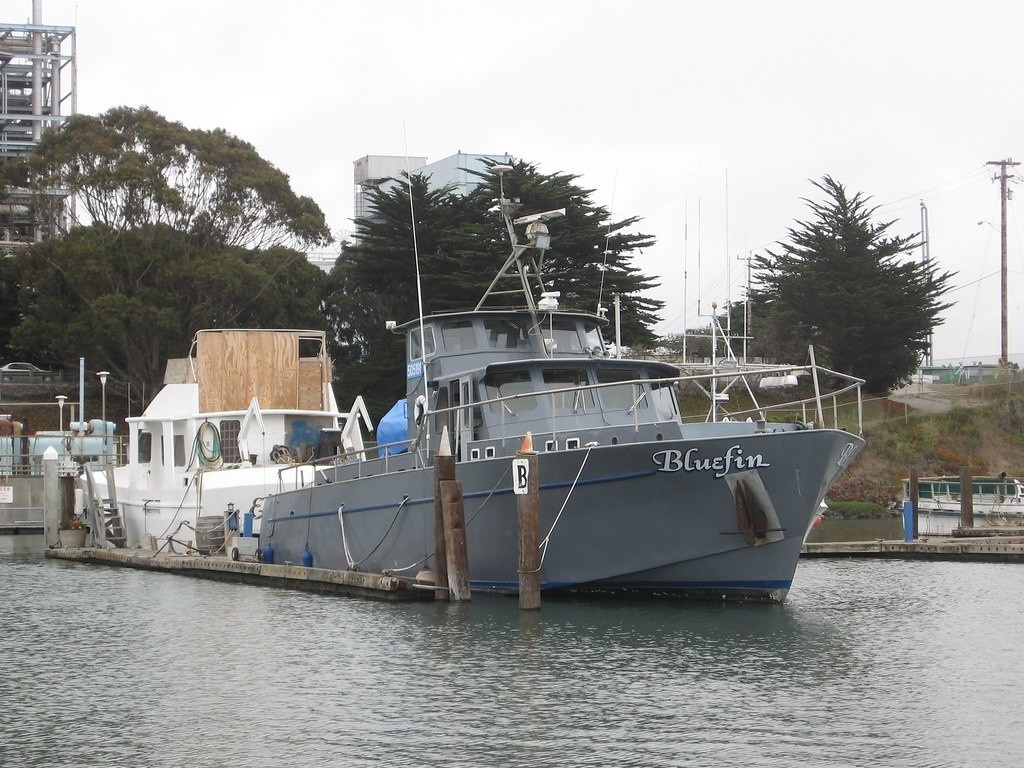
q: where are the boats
[65,161,870,604]
[0,412,117,535]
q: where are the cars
[900,475,1024,536]
[0,362,51,383]
